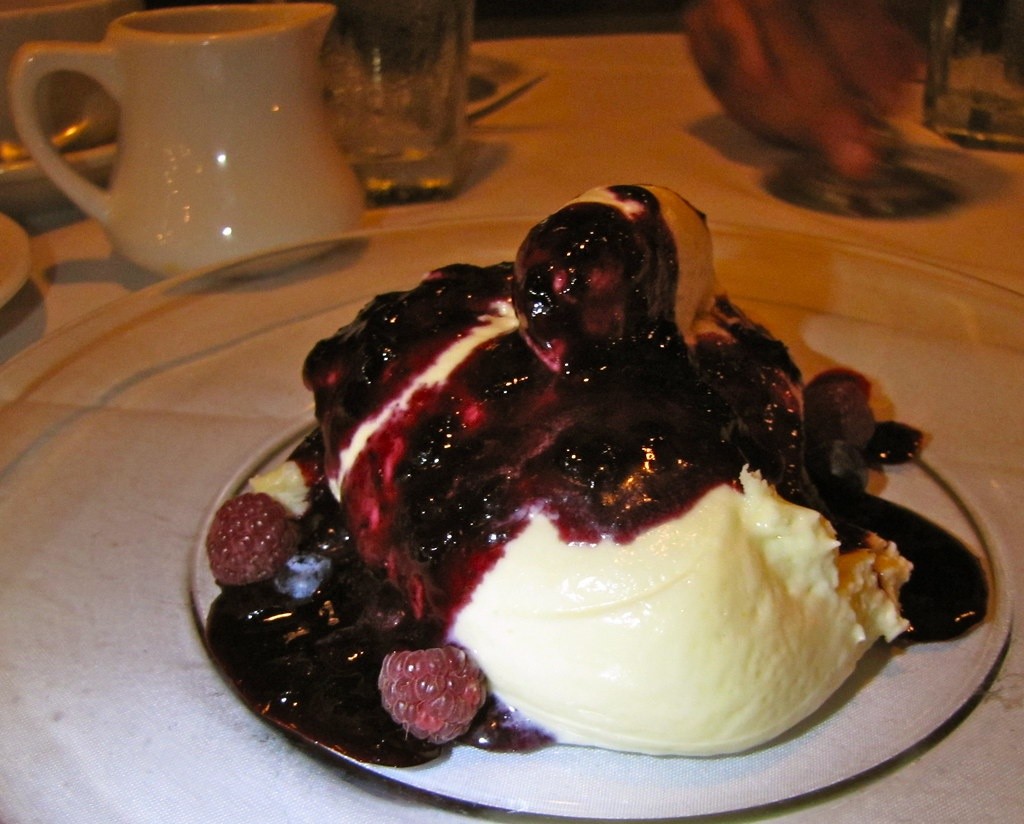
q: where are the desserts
[200,182,992,771]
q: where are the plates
[0,204,33,315]
[1,139,121,213]
[189,391,1024,824]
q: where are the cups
[924,1,1024,154]
[1,0,127,155]
[322,1,485,200]
[5,5,368,281]
